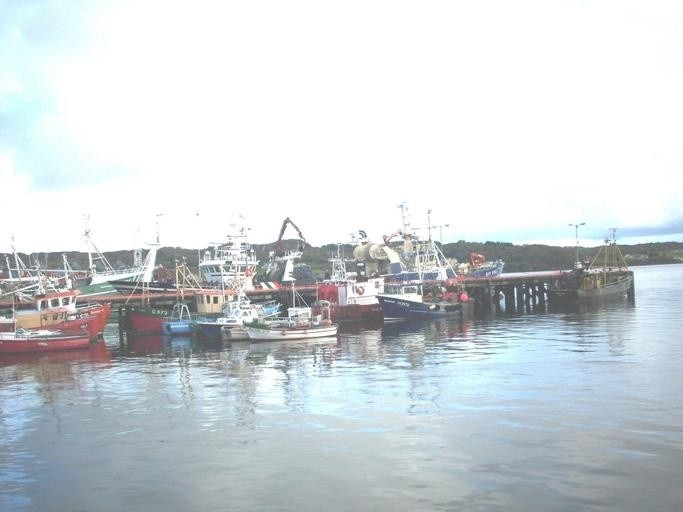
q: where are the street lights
[567,222,586,263]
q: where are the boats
[547,226,636,310]
